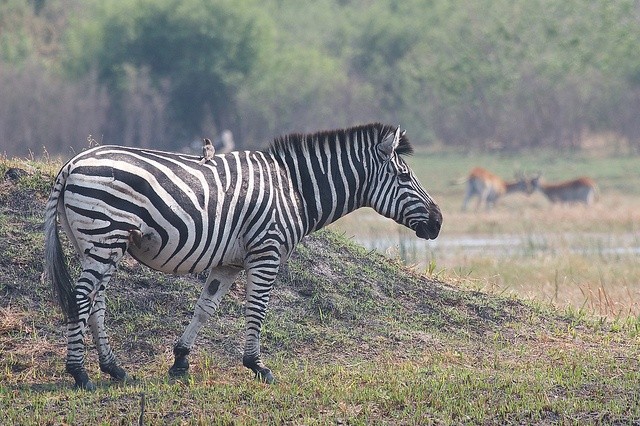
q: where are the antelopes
[531,171,599,208]
[461,167,533,211]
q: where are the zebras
[43,122,444,393]
